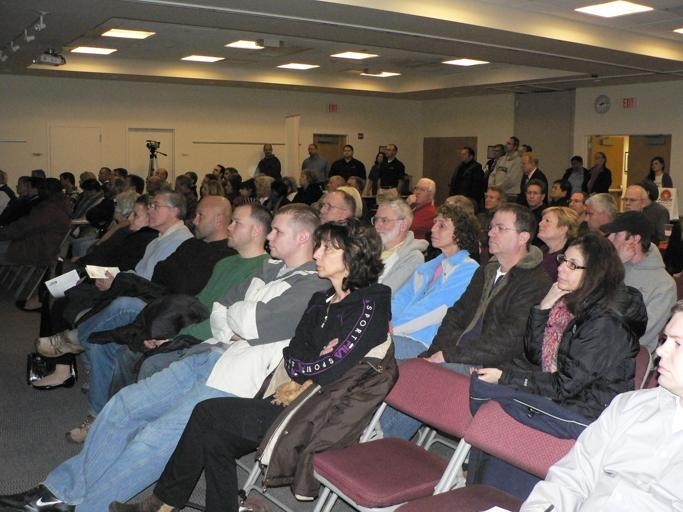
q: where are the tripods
[147,153,160,176]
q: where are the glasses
[415,186,430,193]
[370,216,403,226]
[489,226,515,232]
[319,203,347,211]
[147,203,168,208]
[557,254,586,270]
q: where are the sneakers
[81,370,90,392]
[0,486,75,512]
[35,330,85,358]
[66,416,95,443]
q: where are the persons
[1,137,683,510]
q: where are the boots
[110,495,179,512]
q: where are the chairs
[0,224,73,304]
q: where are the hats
[600,211,652,233]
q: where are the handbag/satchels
[28,353,78,387]
[460,371,591,506]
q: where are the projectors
[35,48,65,67]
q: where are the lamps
[0,10,48,65]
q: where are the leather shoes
[16,300,41,312]
[34,376,74,390]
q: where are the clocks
[594,94,610,114]
